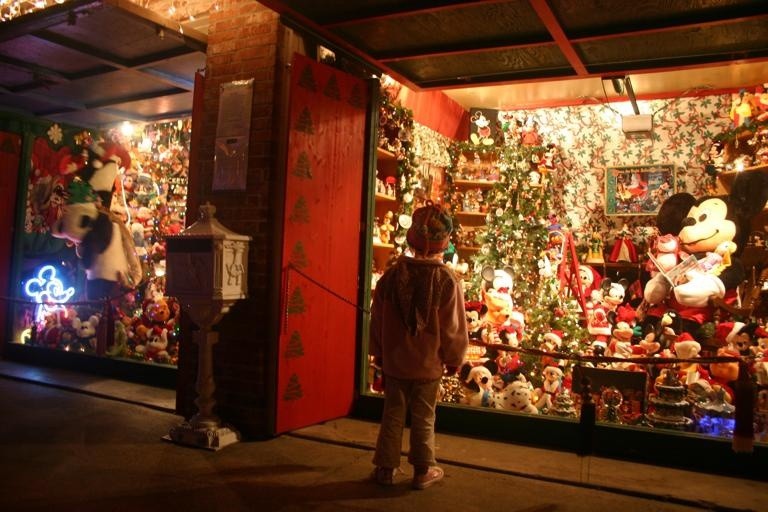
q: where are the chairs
[375,466,396,485]
[411,466,444,490]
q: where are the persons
[367,203,471,490]
[367,203,469,490]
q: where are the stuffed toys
[22,113,194,369]
[368,76,767,442]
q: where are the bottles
[406,204,453,255]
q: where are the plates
[372,147,547,258]
